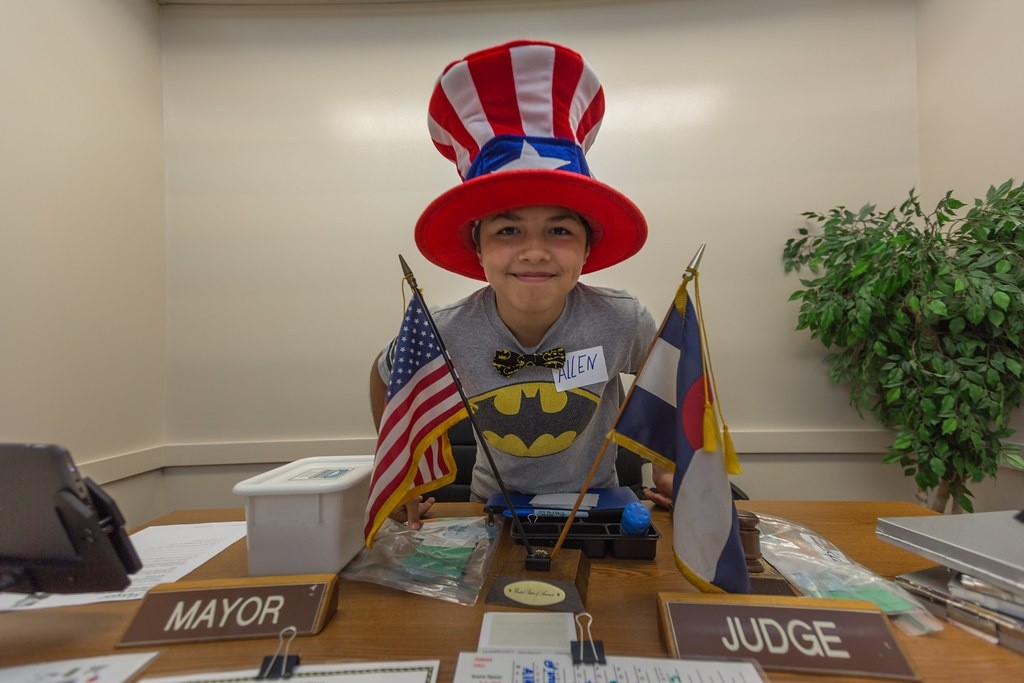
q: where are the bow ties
[494,348,566,379]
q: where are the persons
[369,40,674,530]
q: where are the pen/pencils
[403,518,440,526]
[503,508,589,520]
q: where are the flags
[365,294,472,549]
[608,269,750,596]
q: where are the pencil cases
[484,486,640,526]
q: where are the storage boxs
[233,455,377,575]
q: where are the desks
[1,500,1024,683]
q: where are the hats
[414,38,648,275]
